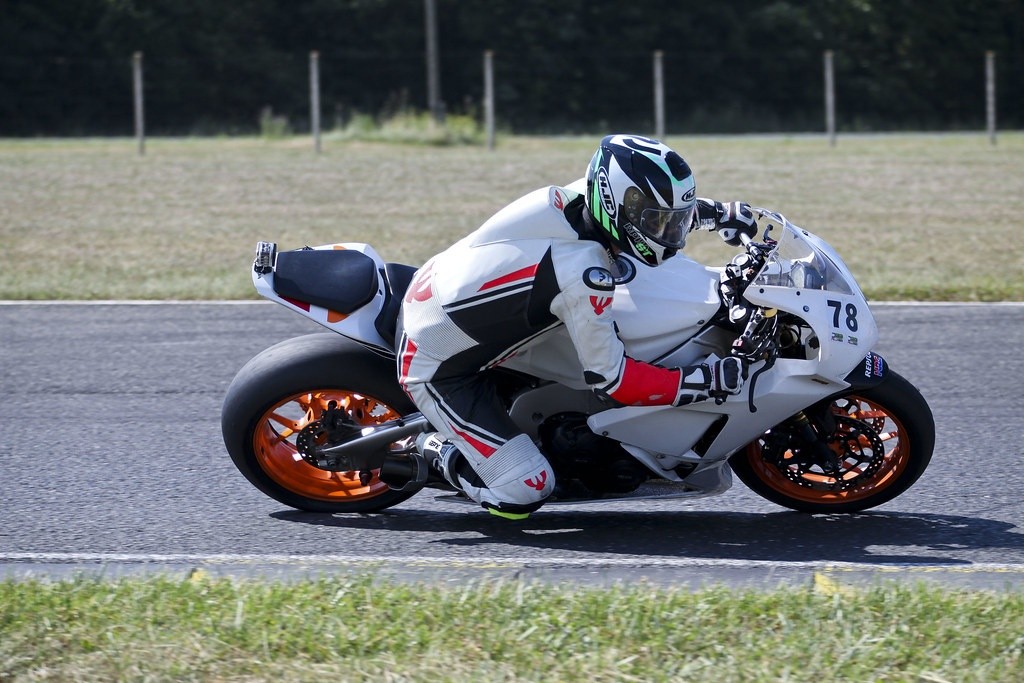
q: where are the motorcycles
[219,206,935,515]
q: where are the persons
[395,135,758,520]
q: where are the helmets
[582,133,697,268]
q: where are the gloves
[671,354,749,408]
[693,195,758,247]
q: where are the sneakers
[413,430,464,493]
